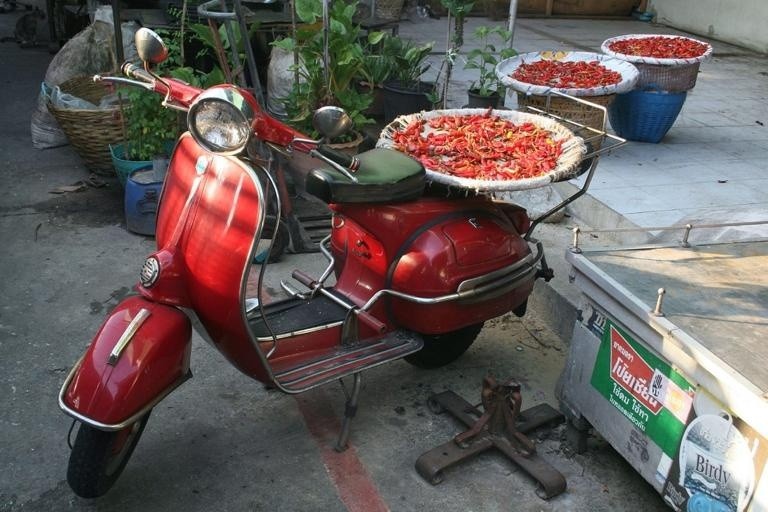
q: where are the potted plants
[111,0,519,238]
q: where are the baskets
[46,74,129,178]
[631,64,700,92]
[606,84,687,144]
[517,92,614,151]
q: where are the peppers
[507,57,623,89]
[391,105,571,180]
[609,37,710,59]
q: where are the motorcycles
[52,25,626,501]
